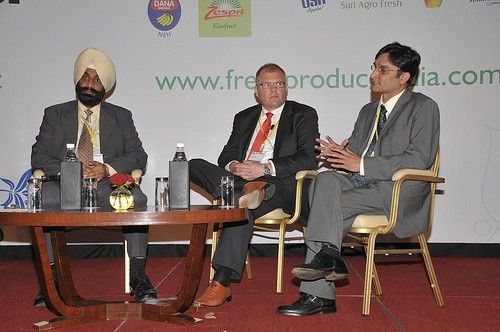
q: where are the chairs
[342,143,445,314]
[210,170,319,294]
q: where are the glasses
[257,81,285,89]
[370,64,402,75]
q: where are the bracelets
[105,166,109,177]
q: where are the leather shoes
[34,279,61,307]
[238,181,276,209]
[130,273,157,301]
[193,280,232,308]
[276,293,336,316]
[290,250,349,281]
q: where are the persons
[31,47,158,305]
[187,63,320,308]
[275,42,441,316]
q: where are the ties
[247,112,274,161]
[351,105,388,188]
[77,109,93,174]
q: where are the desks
[0,206,249,332]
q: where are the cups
[83,178,98,208]
[220,175,234,207]
[155,177,169,207]
[28,178,42,211]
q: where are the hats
[73,47,116,94]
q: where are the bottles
[64,143,78,161]
[173,143,188,161]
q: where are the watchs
[262,163,270,176]
